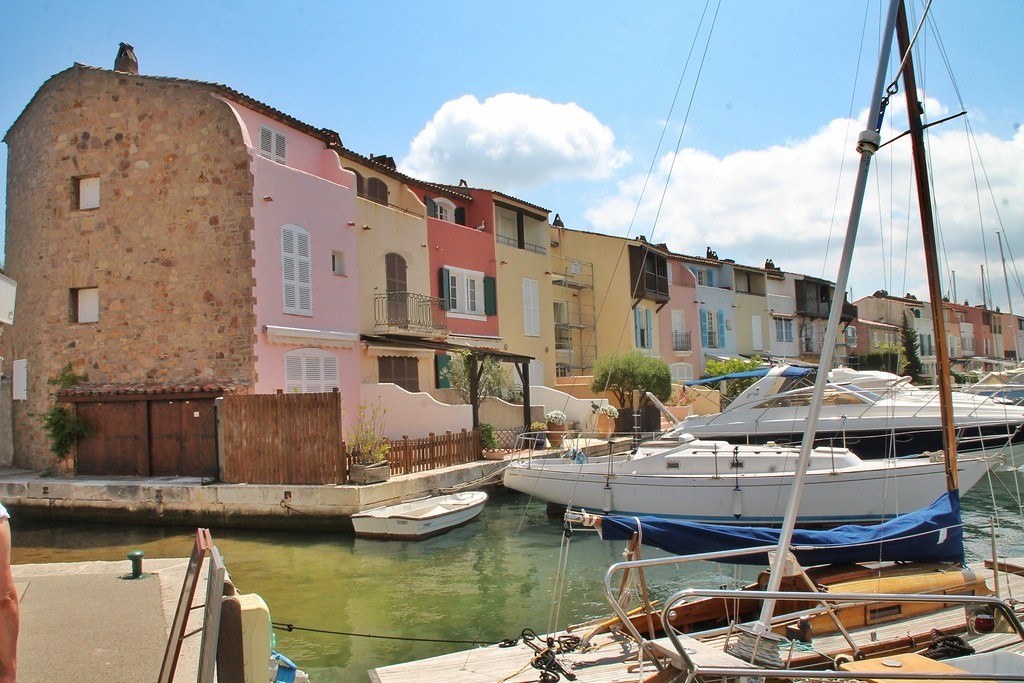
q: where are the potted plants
[345,394,393,485]
[479,422,505,460]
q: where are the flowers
[543,410,566,425]
[530,421,548,431]
[599,404,620,419]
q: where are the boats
[646,366,1024,468]
[349,491,490,541]
[361,0,1024,683]
[502,390,1024,532]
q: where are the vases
[546,421,568,448]
[598,414,616,439]
[533,431,545,449]
